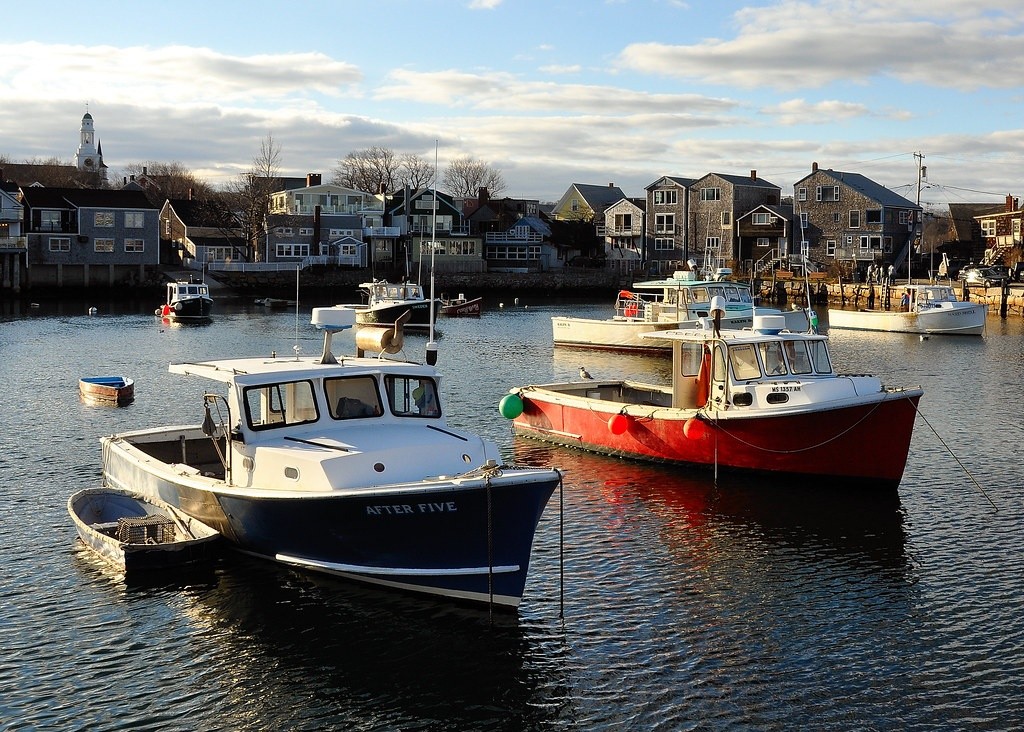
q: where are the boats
[826,240,989,336]
[77,375,135,402]
[265,297,288,309]
[355,218,443,335]
[160,258,215,324]
[508,297,925,497]
[436,292,482,317]
[98,138,565,615]
[67,486,224,579]
[551,235,816,355]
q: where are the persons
[866,264,897,286]
[902,289,910,312]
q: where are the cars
[962,268,1011,288]
[957,263,1024,287]
